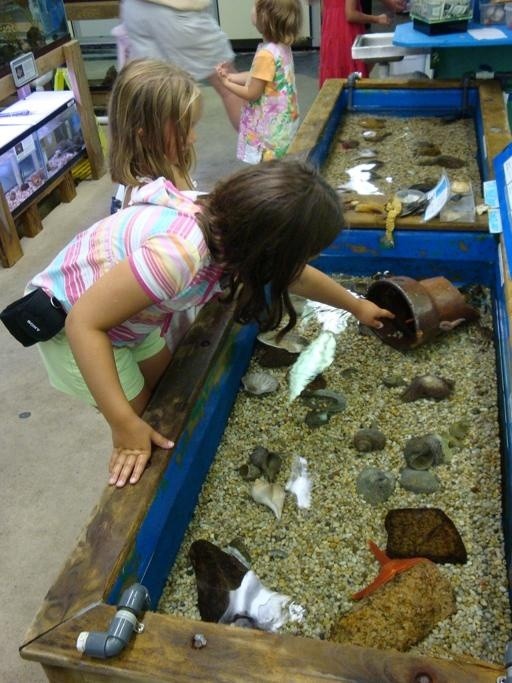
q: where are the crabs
[256,330,310,368]
[241,372,279,397]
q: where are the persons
[317,0,407,89]
[107,56,207,355]
[215,0,303,167]
[22,158,397,490]
[111,0,244,134]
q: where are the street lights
[1,287,67,348]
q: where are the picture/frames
[392,20,512,49]
